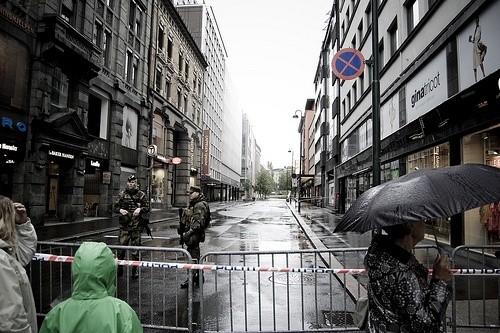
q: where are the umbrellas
[332,163,500,253]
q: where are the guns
[132,201,154,240]
[177,208,190,256]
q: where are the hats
[187,186,201,193]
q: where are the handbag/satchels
[355,296,368,331]
[140,210,150,227]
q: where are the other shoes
[132,269,139,277]
[179,275,204,288]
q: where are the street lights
[293,109,303,214]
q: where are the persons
[39,241,143,333]
[0,195,38,333]
[363,220,453,333]
[179,186,210,290]
[113,175,149,279]
[469,15,485,84]
